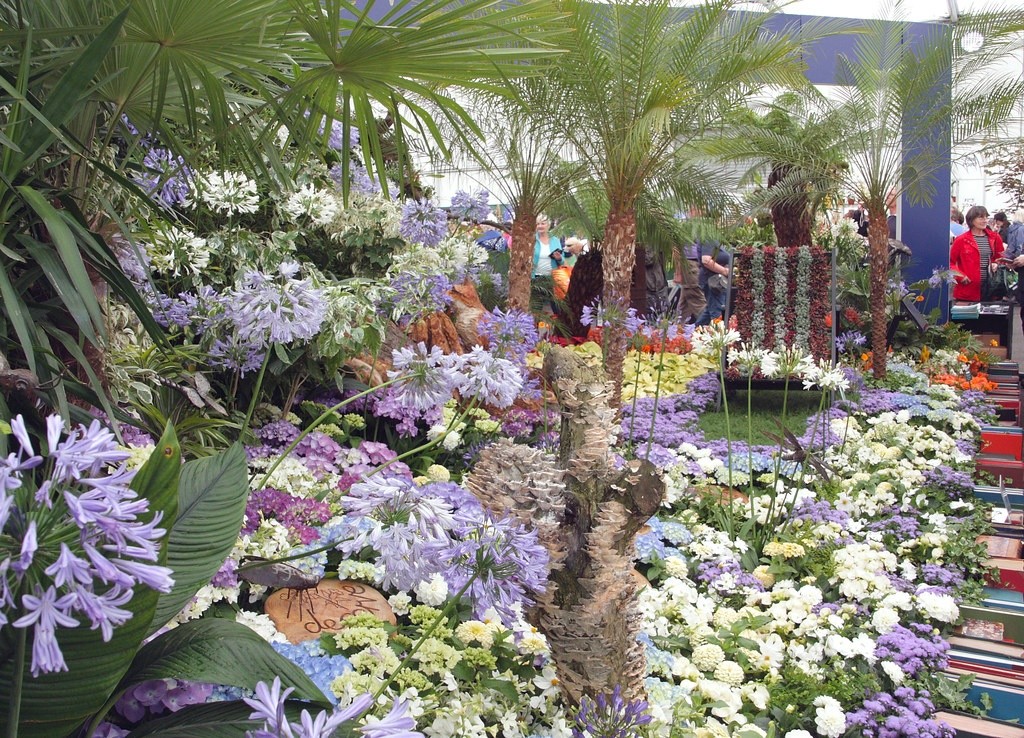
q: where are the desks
[949,300,1013,361]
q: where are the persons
[530,211,589,297]
[854,185,1024,332]
[674,208,731,326]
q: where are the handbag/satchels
[987,258,1019,299]
[550,263,574,301]
[709,273,729,295]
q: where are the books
[930,335,1024,738]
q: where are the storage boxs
[969,334,1008,361]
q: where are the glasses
[977,215,989,219]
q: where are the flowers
[0,109,1006,737]
